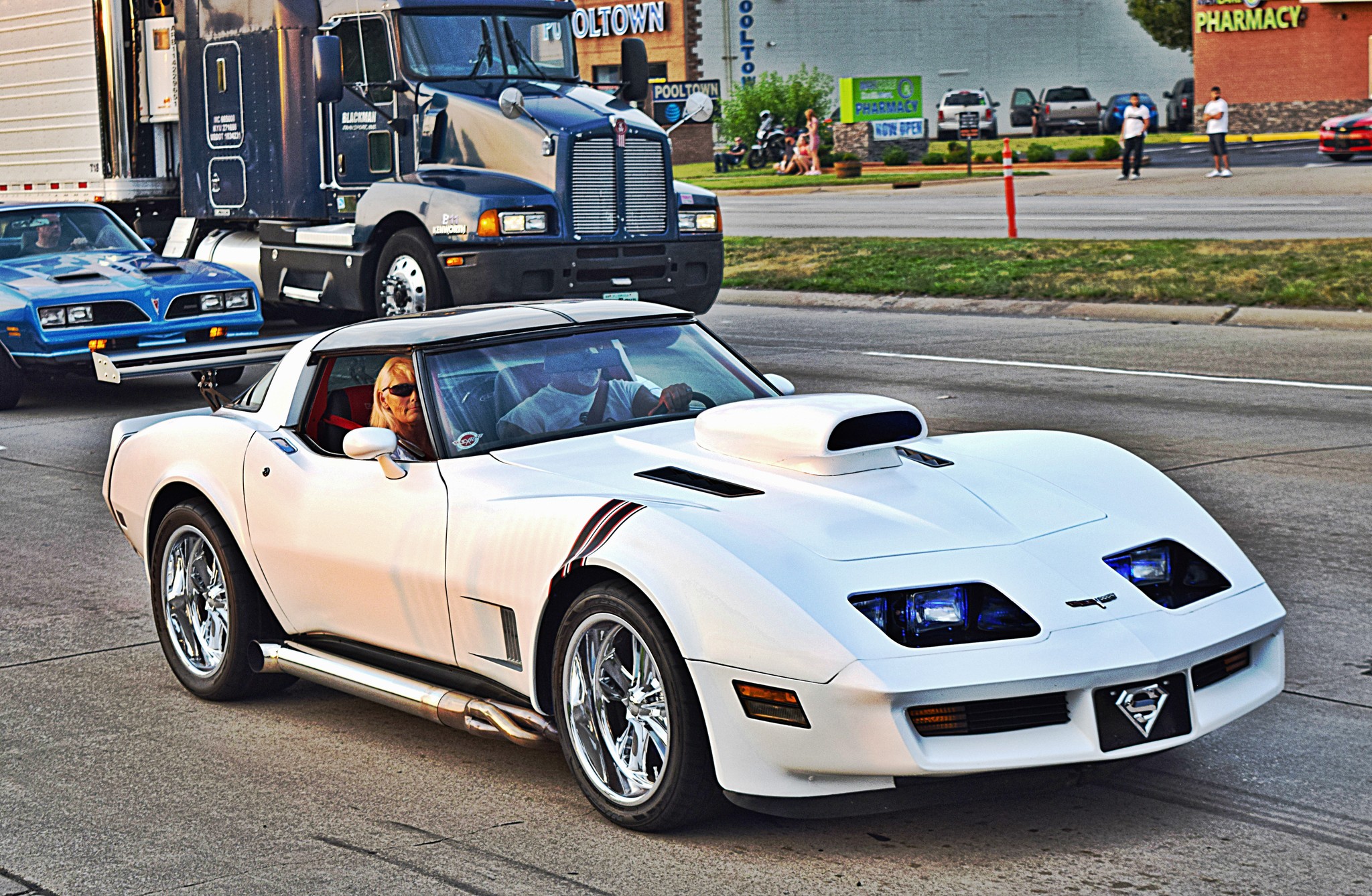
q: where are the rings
[686,388,692,392]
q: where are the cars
[0,202,263,410]
[1102,93,1159,134]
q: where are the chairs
[492,362,551,424]
[18,230,40,251]
[316,383,377,457]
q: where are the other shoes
[1116,174,1126,180]
[1204,168,1232,178]
[1129,173,1140,180]
[805,169,822,176]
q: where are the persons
[19,214,88,257]
[371,357,462,464]
[496,341,693,439]
[775,108,821,175]
[714,135,748,174]
[1203,85,1232,178]
[1117,93,1151,182]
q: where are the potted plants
[830,150,869,178]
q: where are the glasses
[38,221,63,227]
[735,139,741,143]
[382,383,420,397]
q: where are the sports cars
[102,301,1285,833]
[1317,106,1372,161]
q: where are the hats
[759,109,770,117]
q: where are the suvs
[1163,78,1195,132]
[935,87,1001,141]
[1010,85,1102,138]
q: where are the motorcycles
[746,127,806,169]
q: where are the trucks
[0,0,724,337]
